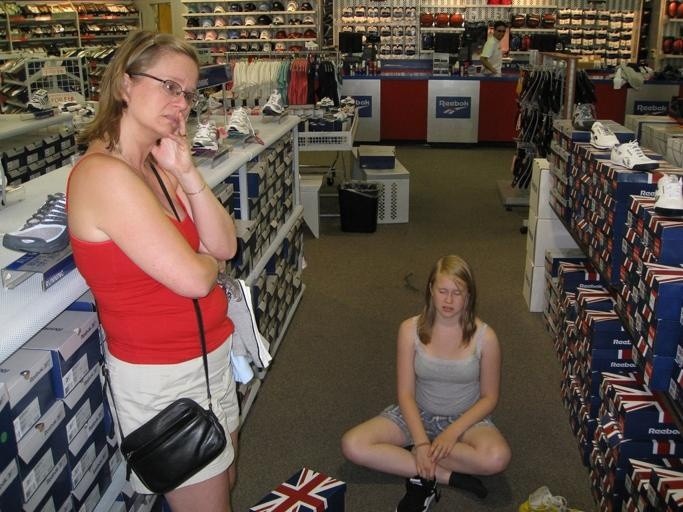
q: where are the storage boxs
[249,467,347,512]
[0,288,162,512]
[0,128,77,192]
[208,128,303,416]
[358,145,396,170]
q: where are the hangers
[228,50,332,62]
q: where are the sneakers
[3,190,70,253]
[226,109,248,137]
[574,103,597,130]
[262,90,285,113]
[28,89,53,110]
[338,106,354,113]
[395,473,441,511]
[317,97,334,106]
[341,97,355,104]
[654,174,683,215]
[192,88,232,112]
[610,140,658,171]
[192,120,217,147]
[589,119,618,151]
[518,486,581,512]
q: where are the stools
[298,174,324,239]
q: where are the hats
[183,1,317,52]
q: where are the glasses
[128,70,199,110]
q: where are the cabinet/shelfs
[0,112,73,146]
[0,1,143,115]
[0,113,307,512]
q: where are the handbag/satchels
[121,397,227,495]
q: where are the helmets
[421,13,463,26]
[512,14,555,27]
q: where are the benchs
[350,146,410,223]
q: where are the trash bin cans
[337,182,380,233]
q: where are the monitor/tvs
[530,34,556,51]
[339,31,362,54]
[434,32,459,53]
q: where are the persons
[478,20,507,74]
[339,251,514,498]
[66,26,244,512]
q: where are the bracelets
[414,442,431,449]
[184,182,206,194]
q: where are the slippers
[510,69,563,190]
[575,71,597,102]
